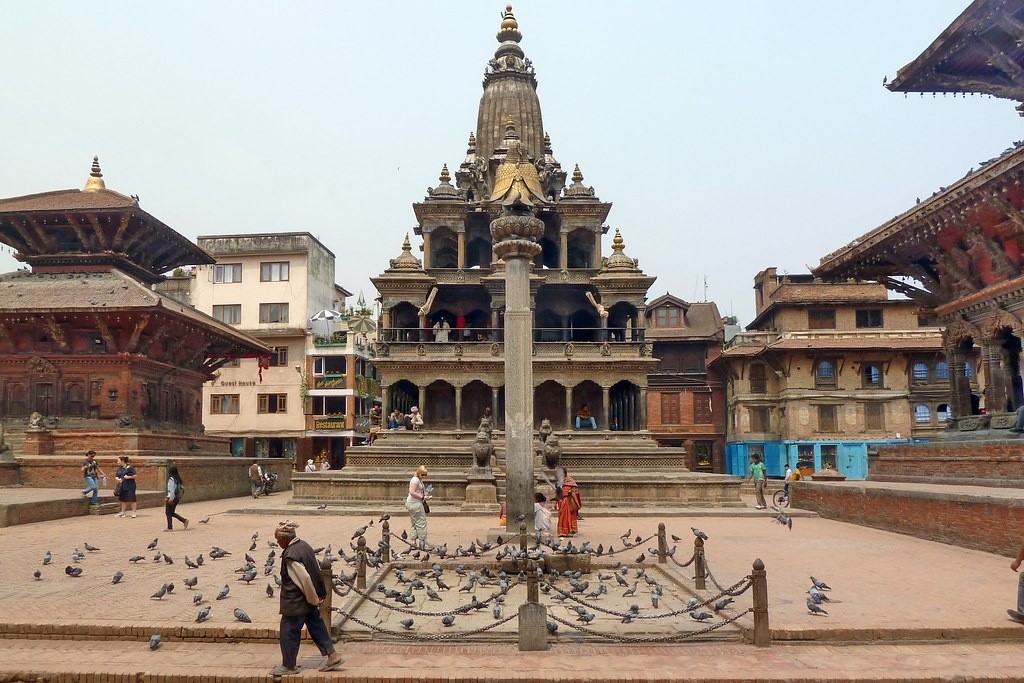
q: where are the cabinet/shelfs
[798,453,837,470]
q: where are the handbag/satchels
[113,482,123,496]
[423,502,430,513]
[499,515,507,526]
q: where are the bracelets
[421,496,424,501]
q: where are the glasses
[420,473,427,477]
[91,456,94,457]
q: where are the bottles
[103,477,107,486]
[425,483,432,495]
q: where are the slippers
[318,658,345,671]
[269,665,300,675]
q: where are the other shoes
[117,512,126,518]
[574,428,579,431]
[252,496,256,499]
[162,528,173,532]
[593,428,599,430]
[184,519,189,529]
[90,502,100,505]
[132,512,137,517]
[81,491,87,498]
[253,493,259,498]
[390,428,394,430]
[399,428,406,431]
[410,546,417,549]
[1007,609,1024,623]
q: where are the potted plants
[313,333,348,344]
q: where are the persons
[320,458,330,471]
[786,461,806,496]
[432,316,452,342]
[305,460,317,473]
[1007,544,1024,627]
[269,519,344,680]
[80,450,109,506]
[745,454,768,509]
[247,462,264,498]
[360,404,425,448]
[783,464,792,498]
[405,465,433,546]
[499,465,580,540]
[162,462,189,532]
[574,405,597,431]
[115,454,138,518]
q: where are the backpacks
[169,477,185,499]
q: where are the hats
[307,459,313,465]
[411,406,418,413]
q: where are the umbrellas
[347,316,376,337]
[309,309,344,340]
[357,290,366,310]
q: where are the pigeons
[688,611,714,620]
[8,266,148,306]
[665,545,677,557]
[769,504,792,530]
[620,528,632,539]
[648,548,659,556]
[492,603,501,620]
[32,514,662,652]
[691,526,709,540]
[805,590,829,615]
[671,535,682,542]
[636,535,642,542]
[714,597,736,612]
[809,576,831,590]
[181,307,184,312]
[399,619,415,630]
[515,511,528,522]
[198,517,211,524]
[547,620,558,632]
[317,504,328,509]
[687,594,698,608]
[442,615,456,626]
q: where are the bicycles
[772,489,790,509]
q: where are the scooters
[257,467,279,496]
